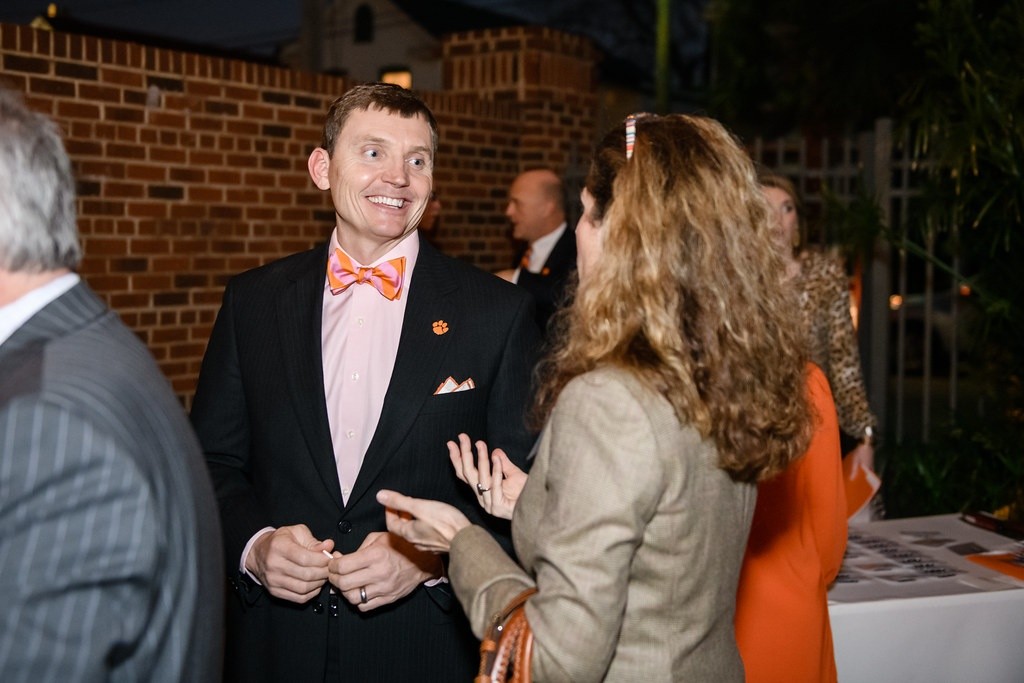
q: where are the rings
[359,586,368,605]
[476,482,491,496]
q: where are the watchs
[858,425,874,443]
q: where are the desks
[827,511,1024,683]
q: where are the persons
[374,112,811,683]
[1,87,227,683]
[752,169,879,529]
[725,357,846,683]
[188,82,531,683]
[414,181,444,244]
[487,169,583,304]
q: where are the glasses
[622,110,662,161]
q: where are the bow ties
[326,248,405,301]
[519,246,533,266]
